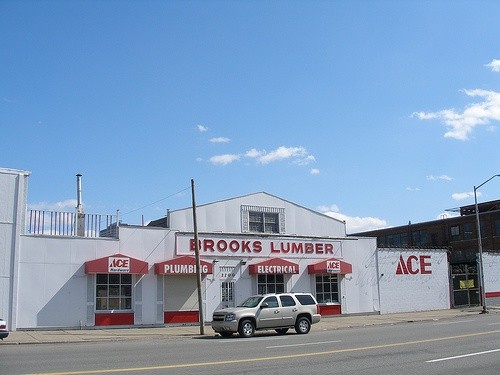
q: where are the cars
[0,319,9,340]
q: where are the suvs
[211,292,321,338]
